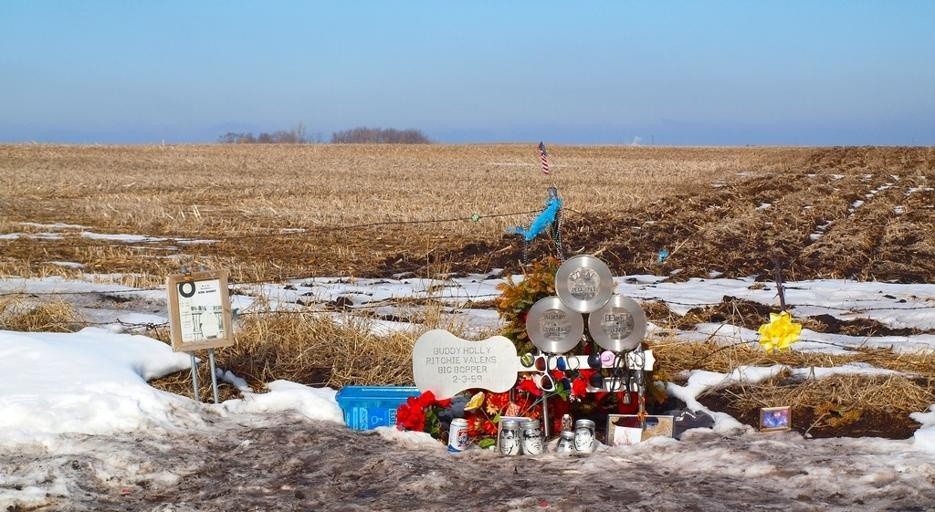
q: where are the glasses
[533,351,632,405]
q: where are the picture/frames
[760,405,793,433]
[168,270,234,351]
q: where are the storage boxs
[334,382,422,432]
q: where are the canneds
[574,419,596,453]
[499,420,520,456]
[517,421,543,456]
[447,418,470,453]
[556,430,575,453]
